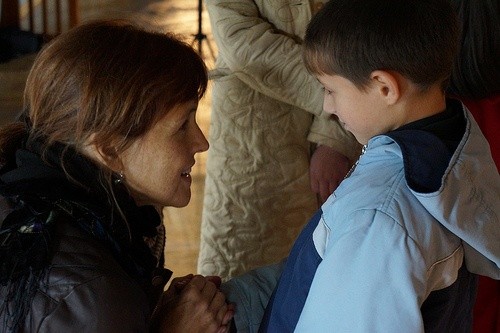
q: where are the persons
[175,0,500,333]
[197,0,363,283]
[0,0,237,333]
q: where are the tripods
[189,0,216,65]
